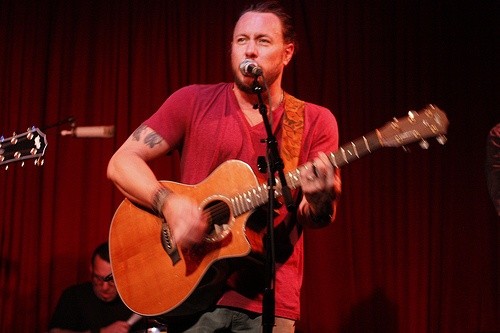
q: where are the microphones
[238,58,263,76]
[61,126,114,138]
[127,314,141,326]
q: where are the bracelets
[151,186,176,215]
[309,204,335,227]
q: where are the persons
[47,241,156,333]
[106,1,342,332]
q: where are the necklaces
[251,101,259,109]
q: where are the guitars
[0,125,49,171]
[108,104,450,319]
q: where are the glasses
[91,271,116,286]
